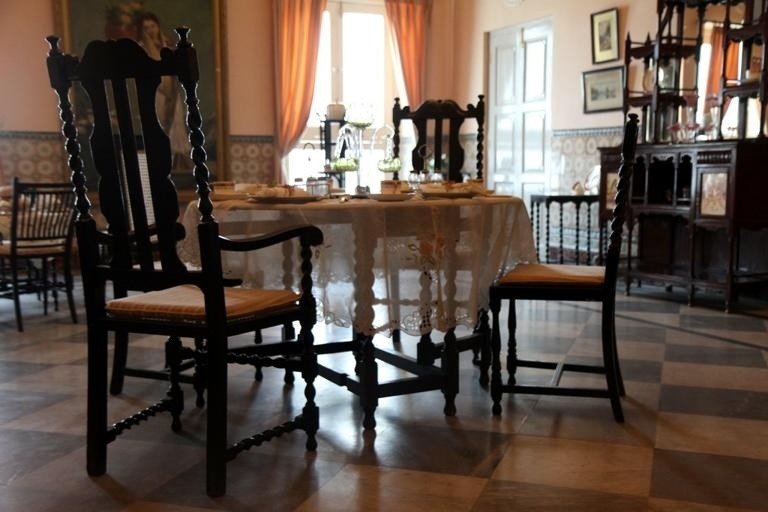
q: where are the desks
[529,191,644,291]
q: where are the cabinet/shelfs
[596,0,768,315]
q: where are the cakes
[380,180,404,196]
[213,182,235,195]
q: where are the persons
[135,13,190,173]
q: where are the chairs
[43,25,324,501]
[107,125,265,412]
[483,110,641,424]
[362,92,488,368]
[0,173,82,334]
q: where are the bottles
[407,169,443,190]
[294,176,329,197]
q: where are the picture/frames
[589,5,620,65]
[50,0,231,211]
[580,63,627,113]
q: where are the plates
[367,193,415,202]
[247,196,321,202]
[421,192,477,198]
